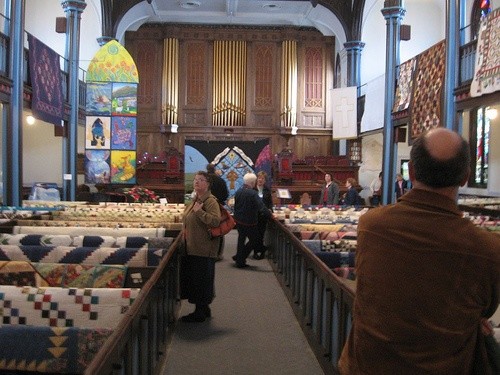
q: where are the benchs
[0,200,186,375]
[272,204,500,375]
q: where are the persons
[253,171,274,259]
[319,173,340,204]
[343,177,358,205]
[336,128,500,375]
[370,172,383,205]
[181,170,222,321]
[206,164,229,261]
[232,173,278,269]
[395,174,408,201]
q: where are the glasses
[194,178,202,183]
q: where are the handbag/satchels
[202,197,236,240]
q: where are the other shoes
[231,256,245,266]
[254,254,261,259]
[182,312,204,322]
[204,309,210,319]
[260,247,267,258]
